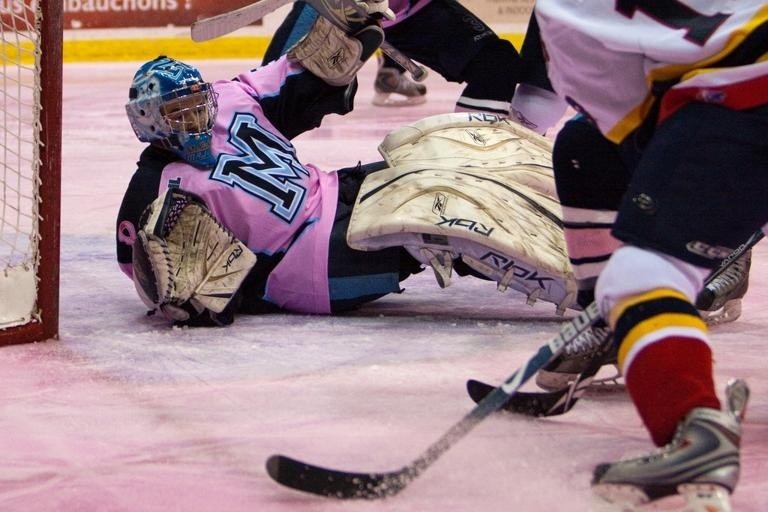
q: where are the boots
[539,327,617,375]
[374,67,426,97]
[697,251,751,310]
[590,408,742,495]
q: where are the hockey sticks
[265,298,606,499]
[466,227,767,416]
[192,1,291,41]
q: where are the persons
[116,1,768,498]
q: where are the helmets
[125,55,218,170]
